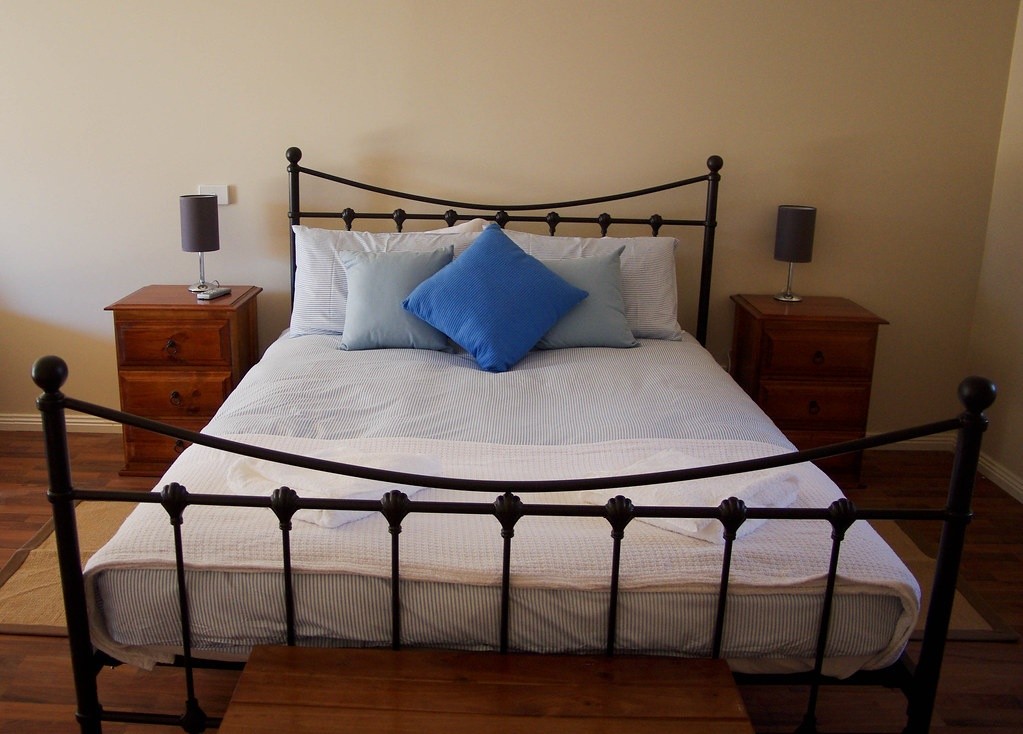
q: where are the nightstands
[103,285,263,478]
[728,292,890,490]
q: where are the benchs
[216,646,757,734]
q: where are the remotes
[197,287,231,299]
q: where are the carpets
[0,500,139,639]
[868,520,1021,644]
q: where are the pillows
[530,245,642,351]
[402,222,589,375]
[483,222,684,342]
[335,244,455,353]
[287,225,486,340]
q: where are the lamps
[179,194,224,293]
[772,204,818,302]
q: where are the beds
[31,147,1001,734]
